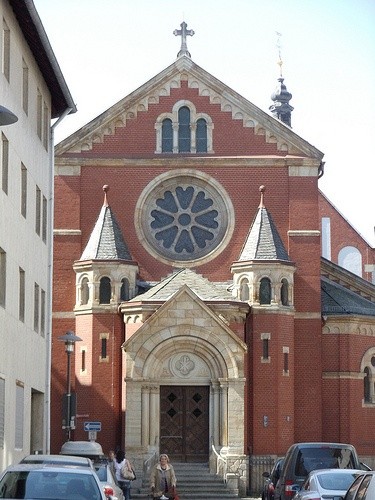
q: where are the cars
[17,454,97,473]
[339,471,375,500]
[0,463,107,500]
[290,469,369,500]
[93,464,128,500]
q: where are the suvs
[261,457,285,500]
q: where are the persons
[105,451,117,471]
[150,454,177,500]
[113,450,133,500]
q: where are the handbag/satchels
[121,466,135,480]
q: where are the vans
[57,441,104,467]
[272,442,360,500]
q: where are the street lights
[55,330,83,443]
